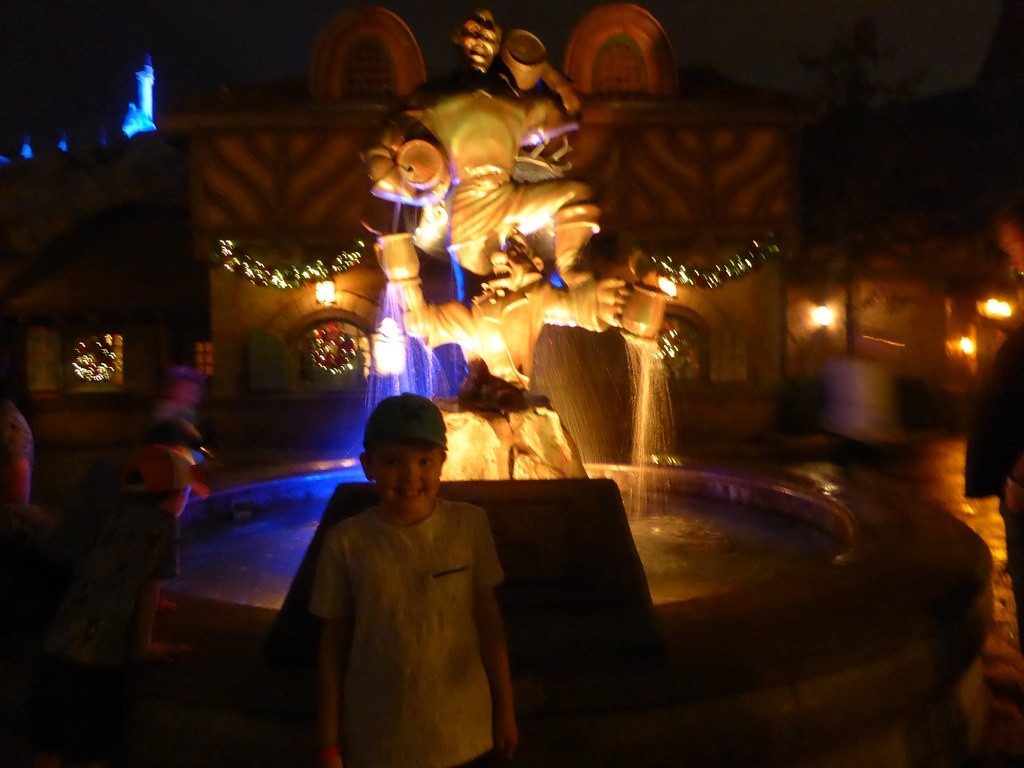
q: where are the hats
[364,392,449,451]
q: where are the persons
[28,415,206,768]
[965,192,1024,655]
[308,393,518,768]
